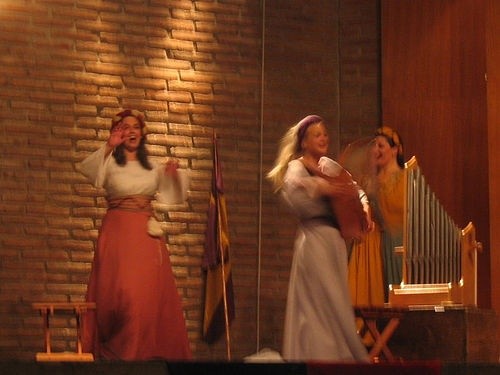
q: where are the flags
[202,135,235,345]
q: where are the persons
[365,127,413,287]
[76,108,193,363]
[268,116,374,360]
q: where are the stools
[354,305,409,364]
[33,301,96,362]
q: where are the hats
[111,109,147,141]
